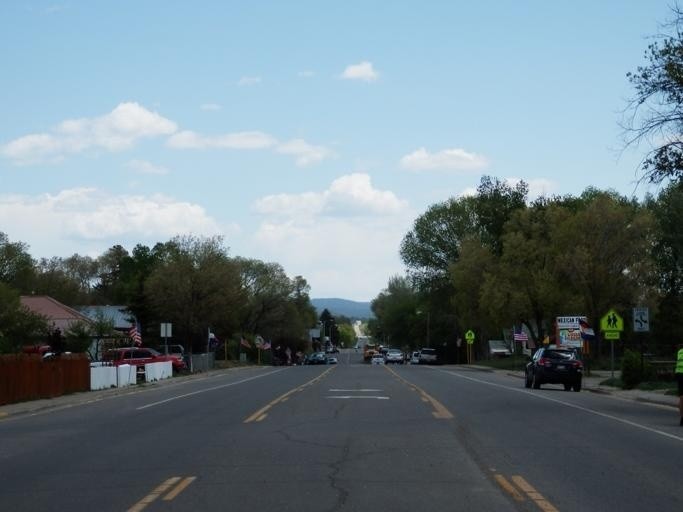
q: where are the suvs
[524,346,582,393]
[488,339,510,360]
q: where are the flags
[240,335,251,349]
[578,318,596,340]
[208,332,218,348]
[128,321,141,347]
[513,330,528,341]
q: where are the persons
[674,344,683,425]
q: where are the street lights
[328,324,337,342]
[323,319,333,336]
[415,308,430,347]
[376,326,389,350]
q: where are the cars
[307,341,339,365]
[22,342,186,379]
[363,343,439,366]
